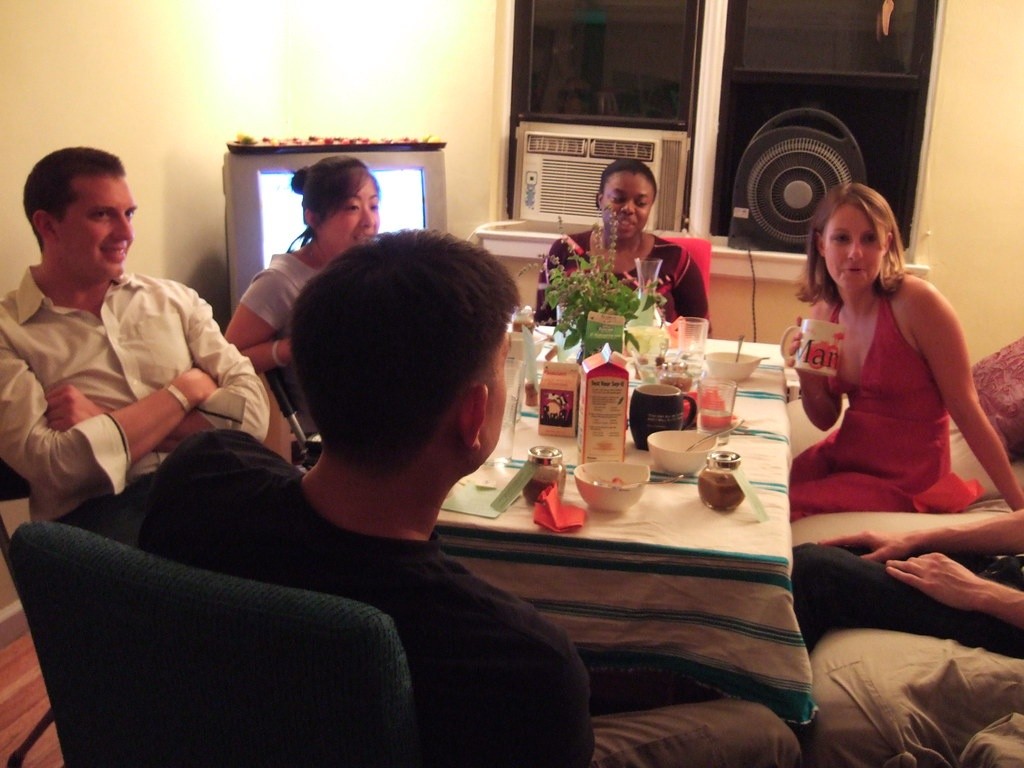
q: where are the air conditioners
[512,120,686,234]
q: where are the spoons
[578,466,683,488]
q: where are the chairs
[9,522,417,768]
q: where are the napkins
[529,481,586,533]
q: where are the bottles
[514,308,538,407]
[554,303,581,364]
[523,446,566,506]
[698,451,746,511]
[660,362,692,392]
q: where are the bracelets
[271,340,289,369]
[801,382,823,401]
[155,383,191,417]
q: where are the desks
[447,341,815,725]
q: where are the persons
[779,183,1024,525]
[226,156,383,466]
[134,228,804,768]
[0,145,270,551]
[535,156,714,349]
[791,507,1024,659]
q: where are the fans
[726,108,865,250]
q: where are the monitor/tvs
[220,150,448,321]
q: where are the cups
[629,383,697,451]
[697,378,737,445]
[781,318,846,376]
[492,360,526,463]
[678,317,709,363]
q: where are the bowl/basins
[648,430,718,473]
[507,332,547,361]
[706,352,761,382]
[573,462,650,513]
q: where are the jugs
[624,259,672,384]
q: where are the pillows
[971,334,1024,466]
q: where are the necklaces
[307,242,315,267]
[632,231,640,253]
